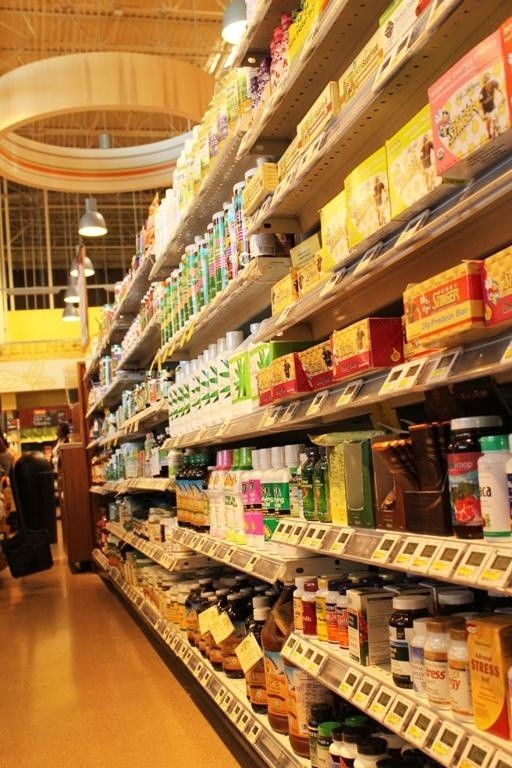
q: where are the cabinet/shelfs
[0,0,512,768]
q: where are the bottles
[174,411,510,767]
[133,367,172,477]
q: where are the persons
[373,176,389,227]
[354,327,367,351]
[322,347,336,370]
[49,422,72,520]
[477,70,503,139]
[282,359,292,378]
[418,134,441,189]
[314,254,324,277]
[404,296,422,324]
[6,438,59,546]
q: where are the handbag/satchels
[3,527,53,579]
